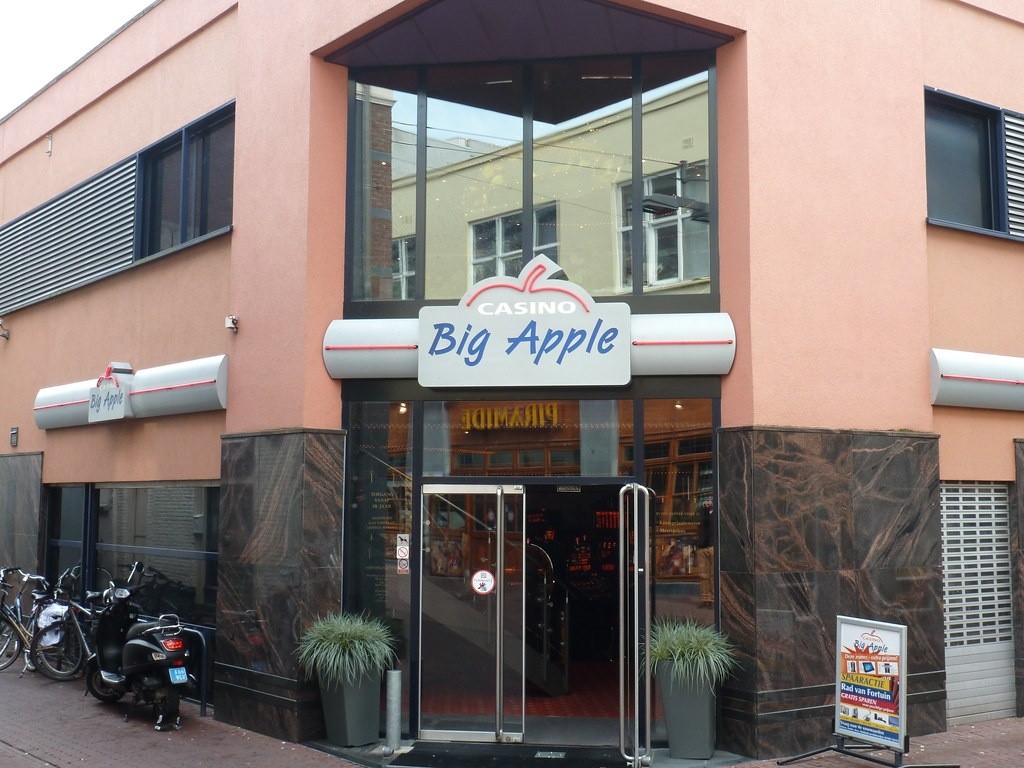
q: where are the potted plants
[289,610,403,747]
[639,612,749,758]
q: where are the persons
[696,498,715,608]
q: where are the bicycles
[0,562,147,683]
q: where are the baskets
[53,580,84,606]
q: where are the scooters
[85,560,190,731]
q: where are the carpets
[379,662,664,719]
